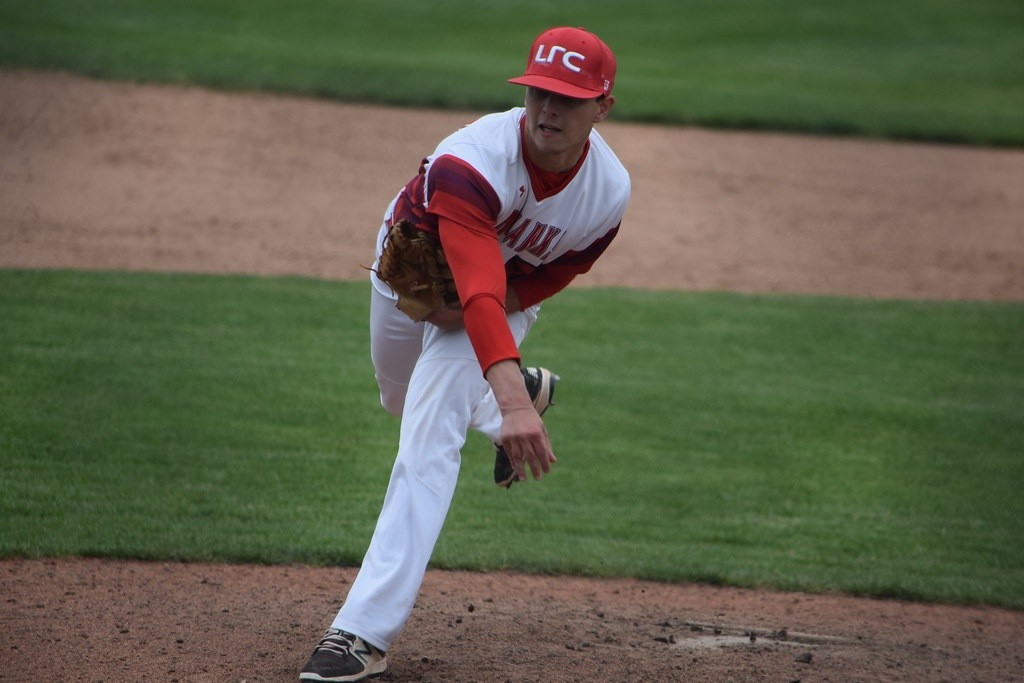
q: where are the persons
[299,26,631,683]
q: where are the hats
[508,26,616,100]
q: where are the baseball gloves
[374,216,458,325]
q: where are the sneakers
[494,365,561,489]
[299,626,388,682]
